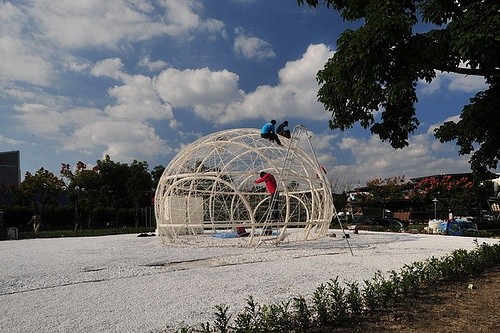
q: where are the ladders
[254,124,356,257]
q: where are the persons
[276,121,291,139]
[253,171,279,219]
[28,211,42,232]
[260,120,283,146]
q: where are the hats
[284,121,288,126]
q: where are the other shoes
[278,143,283,146]
[271,215,279,219]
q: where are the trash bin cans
[7,227,19,239]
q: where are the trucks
[421,220,478,236]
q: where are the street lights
[432,198,438,218]
